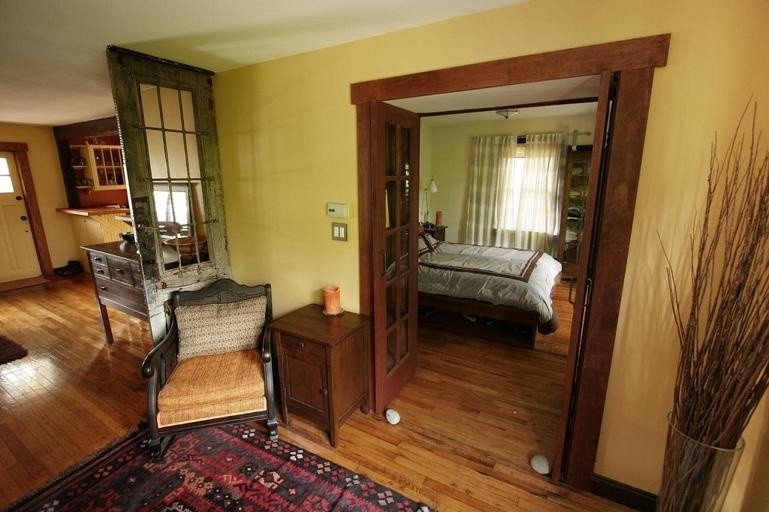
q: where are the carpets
[2,422,431,511]
[1,275,53,293]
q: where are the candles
[322,286,342,314]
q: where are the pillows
[171,294,267,364]
[417,233,437,253]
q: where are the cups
[322,289,340,318]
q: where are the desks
[79,238,209,345]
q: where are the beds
[409,230,564,351]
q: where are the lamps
[495,108,519,121]
[423,177,437,229]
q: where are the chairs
[140,274,282,463]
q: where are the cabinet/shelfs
[557,144,593,280]
[70,212,130,273]
[70,143,126,193]
[266,301,370,449]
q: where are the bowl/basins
[121,234,134,243]
[164,236,207,257]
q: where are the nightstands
[422,224,448,241]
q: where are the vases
[656,409,746,512]
